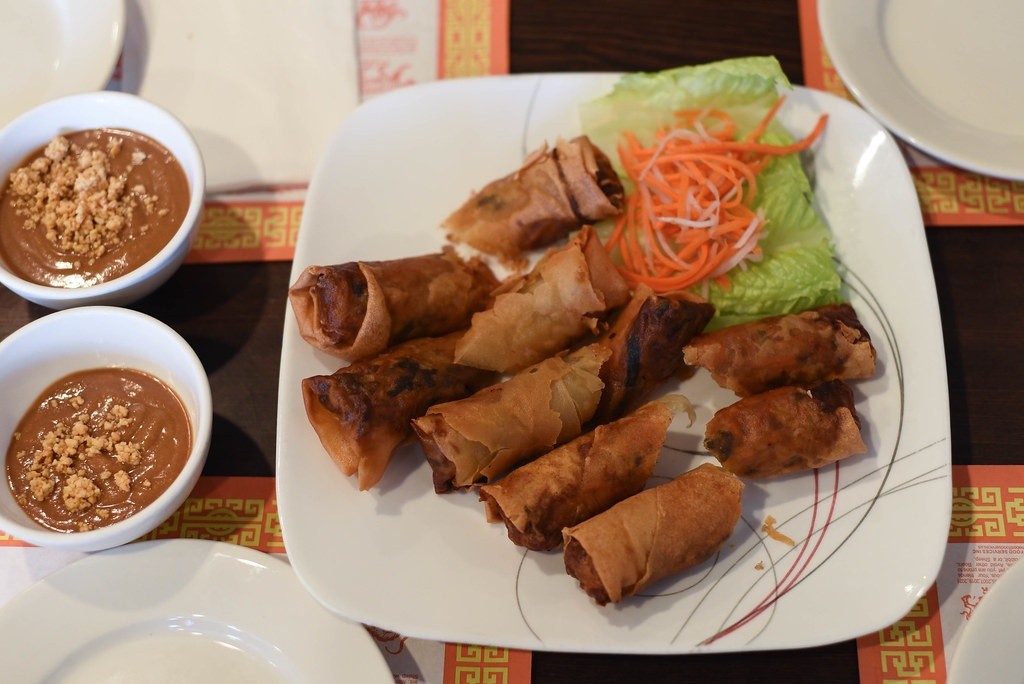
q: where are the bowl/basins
[0,91,207,308]
[0,306,213,552]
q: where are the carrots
[606,90,830,294]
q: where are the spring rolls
[681,303,877,481]
[561,463,746,605]
[290,133,716,552]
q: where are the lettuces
[582,171,843,329]
[583,53,814,202]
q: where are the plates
[946,556,1024,684]
[0,0,125,128]
[0,539,392,684]
[817,0,1024,181]
[279,73,951,654]
